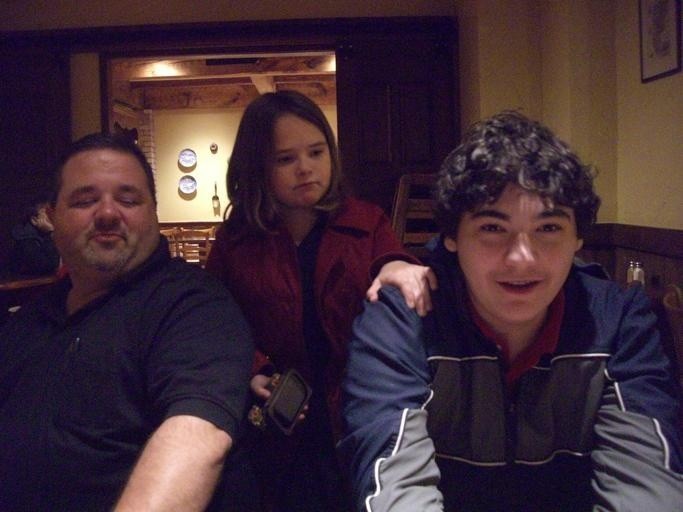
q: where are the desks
[0,270,54,294]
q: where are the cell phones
[262,367,312,437]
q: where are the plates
[177,148,198,167]
[178,175,197,194]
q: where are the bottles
[625,260,646,286]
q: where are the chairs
[157,226,179,259]
[175,226,210,267]
[389,174,444,258]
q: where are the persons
[1,132,263,511]
[205,88,441,511]
[8,190,60,278]
[336,108,682,512]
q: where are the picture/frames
[638,1,682,84]
